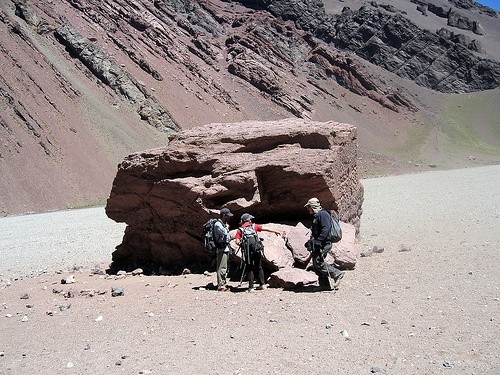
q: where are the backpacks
[239,223,264,265]
[318,211,342,243]
[203,218,225,253]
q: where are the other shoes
[313,284,331,291]
[256,284,267,290]
[334,271,345,286]
[245,287,255,293]
[218,287,230,292]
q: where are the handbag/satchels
[308,240,314,251]
[228,244,245,274]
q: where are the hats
[241,213,254,221]
[304,197,320,208]
[220,208,234,216]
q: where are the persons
[236,213,281,293]
[213,208,236,292]
[303,198,346,292]
